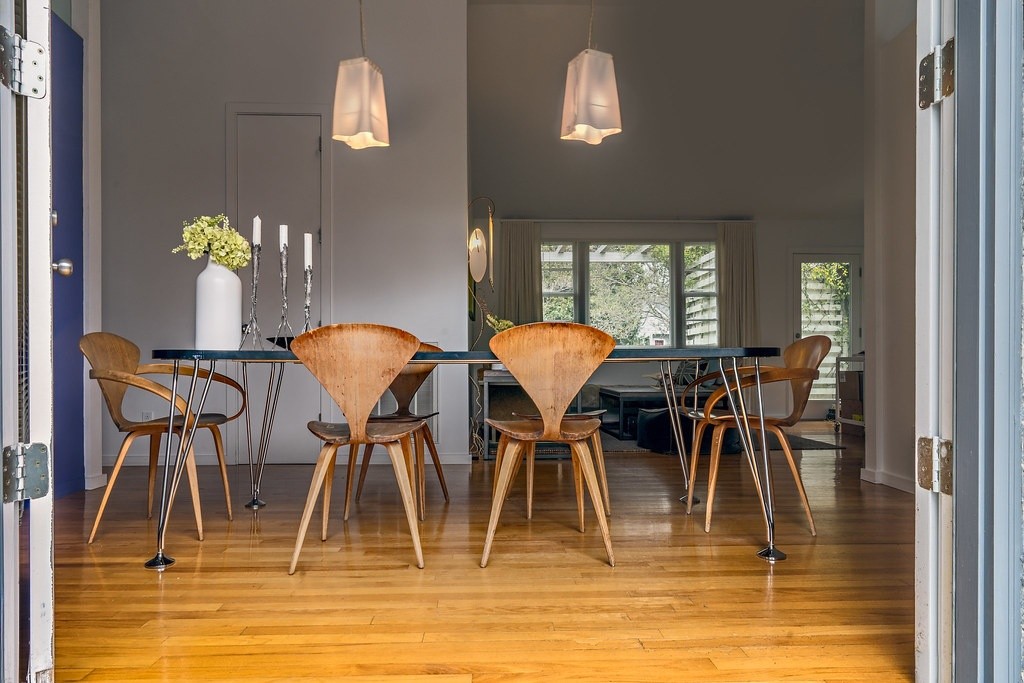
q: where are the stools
[638,408,742,455]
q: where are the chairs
[79,333,246,543]
[681,334,831,536]
[288,324,619,574]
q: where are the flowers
[170,212,252,270]
[485,314,516,332]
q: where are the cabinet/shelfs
[834,357,865,432]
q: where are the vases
[196,252,243,351]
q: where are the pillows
[675,360,709,385]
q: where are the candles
[280,225,288,252]
[304,233,312,271]
[253,215,261,246]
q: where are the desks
[600,384,715,440]
[482,369,582,460]
[152,346,789,562]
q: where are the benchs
[584,358,743,441]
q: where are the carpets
[602,413,846,453]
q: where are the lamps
[332,0,390,150]
[561,0,623,145]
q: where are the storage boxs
[838,370,863,419]
